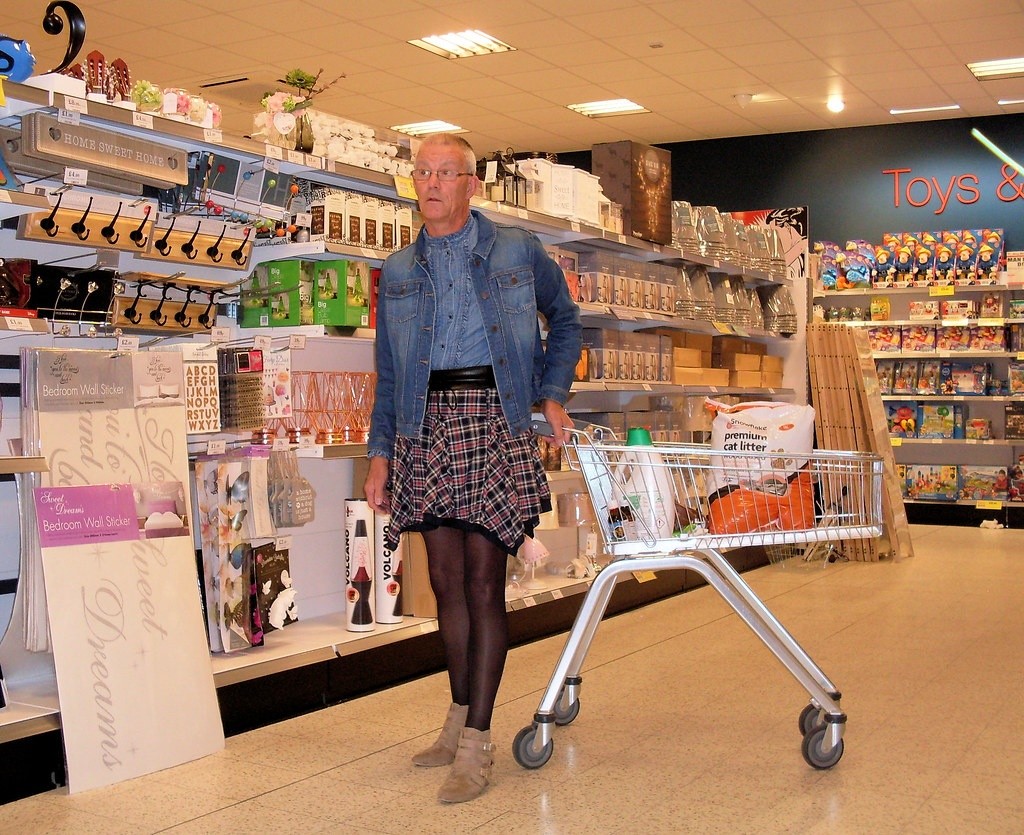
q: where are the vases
[285,67,346,154]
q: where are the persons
[361,132,585,805]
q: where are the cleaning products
[612,428,679,541]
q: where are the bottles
[607,427,676,545]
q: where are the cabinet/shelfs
[813,288,1023,507]
[0,77,813,747]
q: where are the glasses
[411,168,474,181]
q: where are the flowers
[261,89,312,117]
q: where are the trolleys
[510,414,889,769]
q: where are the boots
[412,703,470,768]
[437,726,496,804]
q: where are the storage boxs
[591,140,672,246]
